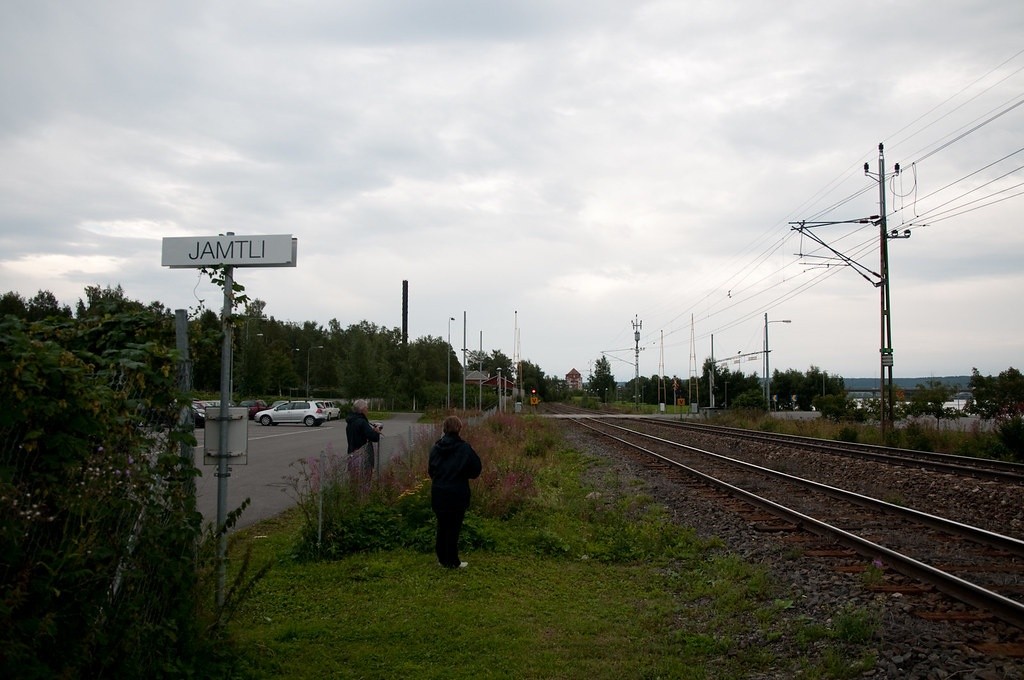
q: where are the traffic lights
[531,387,536,395]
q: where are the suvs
[254,400,329,427]
[313,400,341,421]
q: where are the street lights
[245,318,268,374]
[448,316,456,411]
[289,348,300,396]
[762,319,792,399]
[306,345,324,399]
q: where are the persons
[427,415,482,569]
[346,399,383,511]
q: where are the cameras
[373,424,383,432]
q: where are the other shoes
[438,562,468,568]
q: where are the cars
[270,401,288,410]
[190,399,222,427]
[239,399,270,418]
